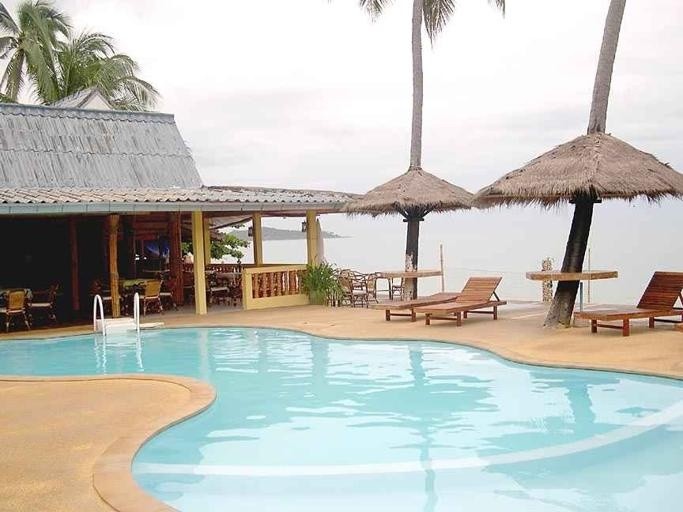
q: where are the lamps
[300,221,307,232]
[247,226,253,237]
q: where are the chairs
[374,290,458,325]
[574,272,682,336]
[412,277,506,324]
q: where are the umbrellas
[316,218,324,266]
